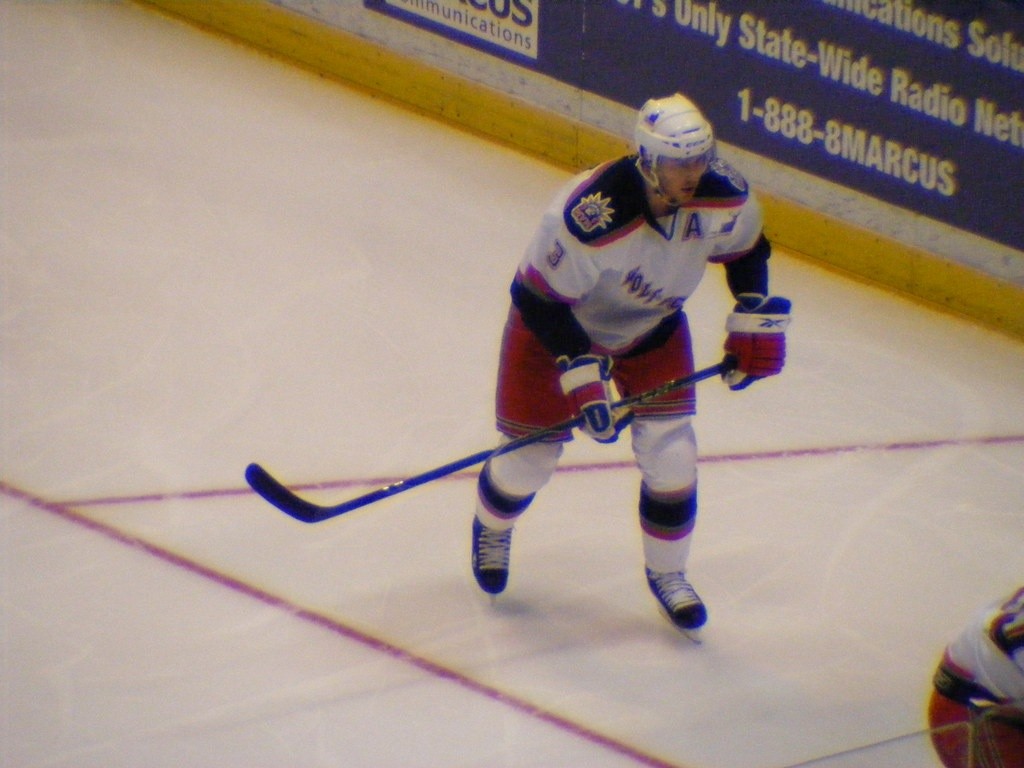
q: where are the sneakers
[469,512,513,603]
[643,566,709,641]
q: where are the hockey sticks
[244,359,735,524]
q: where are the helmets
[633,92,715,161]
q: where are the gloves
[719,312,791,377]
[559,362,615,441]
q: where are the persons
[472,93,791,643]
[927,585,1024,768]
